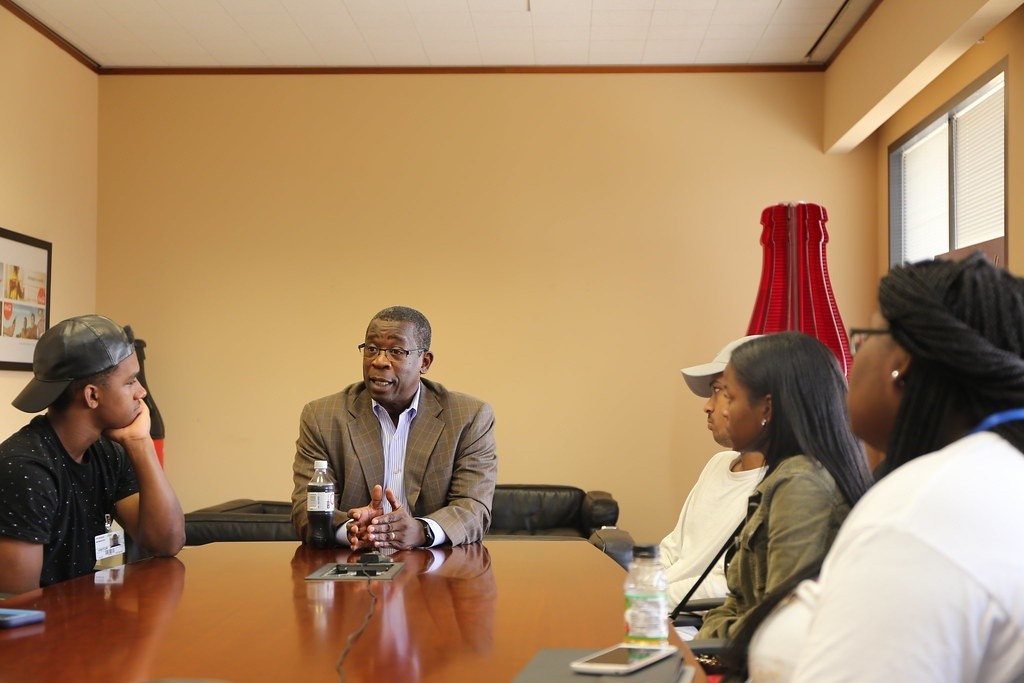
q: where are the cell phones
[569,644,678,675]
[0,608,45,628]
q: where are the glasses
[848,327,891,356]
[358,343,424,362]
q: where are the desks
[0,540,710,683]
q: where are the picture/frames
[0,227,52,372]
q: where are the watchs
[421,520,434,547]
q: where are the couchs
[487,484,635,573]
[183,498,302,547]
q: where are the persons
[746,250,1023,682]
[291,306,497,551]
[654,329,875,671]
[290,542,496,682]
[8,264,26,299]
[110,569,121,579]
[1,314,186,594]
[111,535,119,547]
[16,309,45,340]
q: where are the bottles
[306,460,335,550]
[624,543,669,648]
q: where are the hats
[681,333,766,397]
[11,315,134,412]
